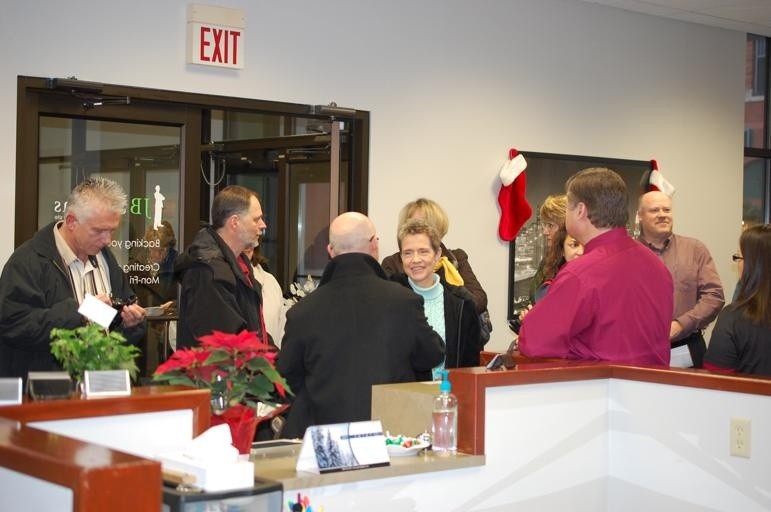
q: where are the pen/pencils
[288,493,324,512]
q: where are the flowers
[154,328,296,416]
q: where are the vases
[208,407,257,454]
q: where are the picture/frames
[504,150,657,326]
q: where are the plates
[385,437,431,456]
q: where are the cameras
[111,294,137,316]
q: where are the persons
[508,167,726,368]
[279,197,492,441]
[1,177,148,385]
[174,186,287,354]
[703,224,770,381]
